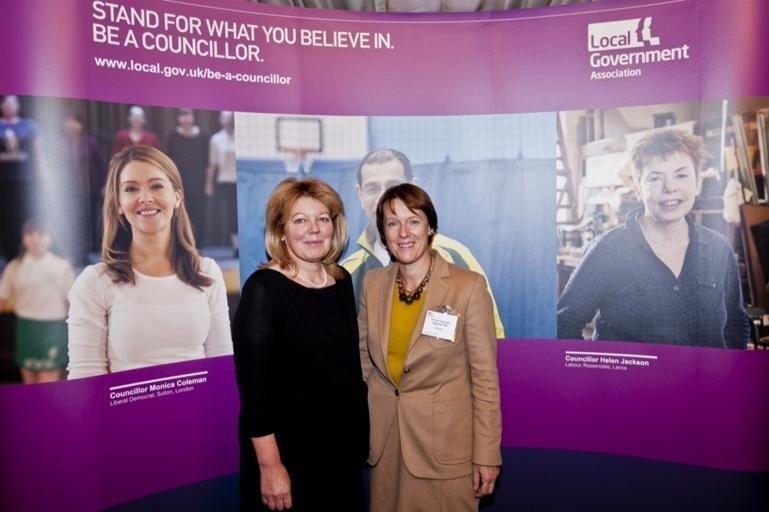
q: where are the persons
[357,184,503,512]
[233,176,369,512]
[558,129,751,349]
[65,145,233,382]
[1,217,75,385]
[109,105,238,243]
[1,95,33,141]
[338,149,506,339]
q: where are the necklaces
[289,263,327,288]
[396,255,432,304]
